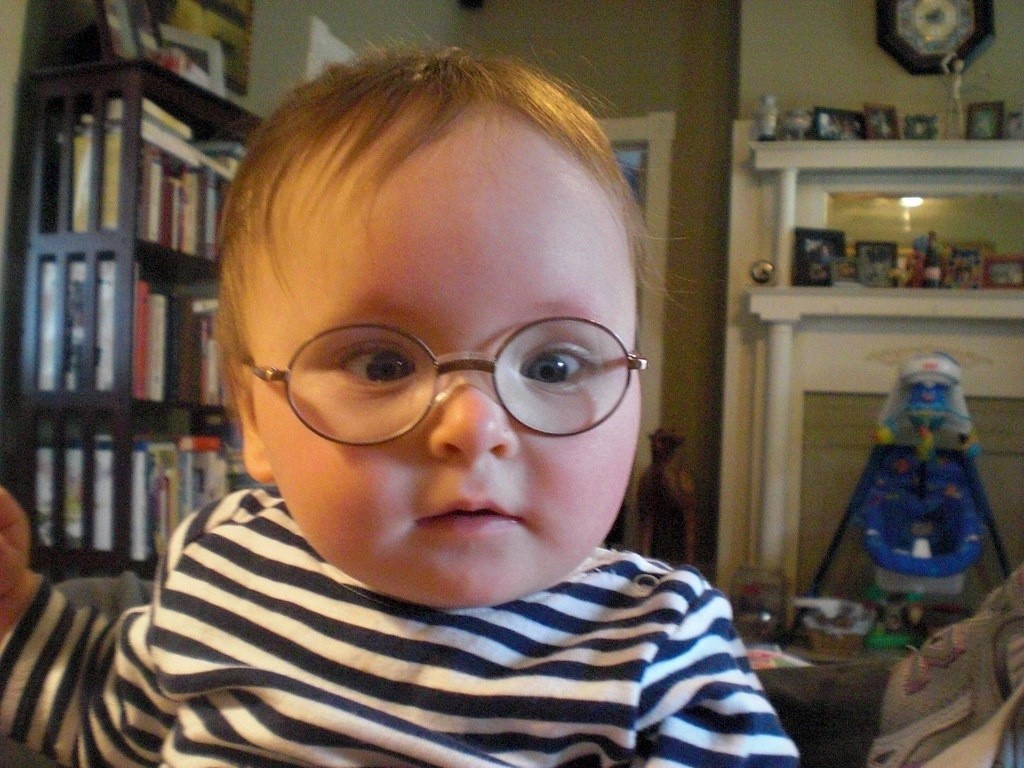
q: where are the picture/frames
[854,241,897,289]
[793,226,846,286]
[981,252,1024,289]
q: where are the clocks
[875,0,998,76]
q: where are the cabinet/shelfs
[13,51,265,586]
[748,139,1024,640]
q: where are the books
[32,94,286,567]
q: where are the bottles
[757,90,778,142]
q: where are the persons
[0,43,804,768]
[943,53,964,139]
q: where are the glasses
[239,314,647,446]
